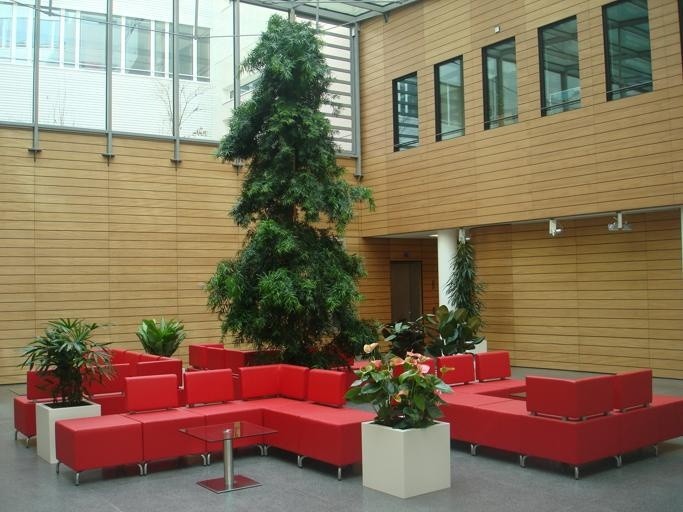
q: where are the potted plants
[441,240,487,384]
[344,325,455,498]
[17,318,119,464]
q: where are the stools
[650,397,683,457]
[433,394,539,467]
[55,414,144,485]
[299,407,377,479]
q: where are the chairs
[119,364,348,474]
[14,349,183,448]
[391,351,526,405]
[522,369,657,479]
[189,344,354,401]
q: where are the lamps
[459,212,633,244]
